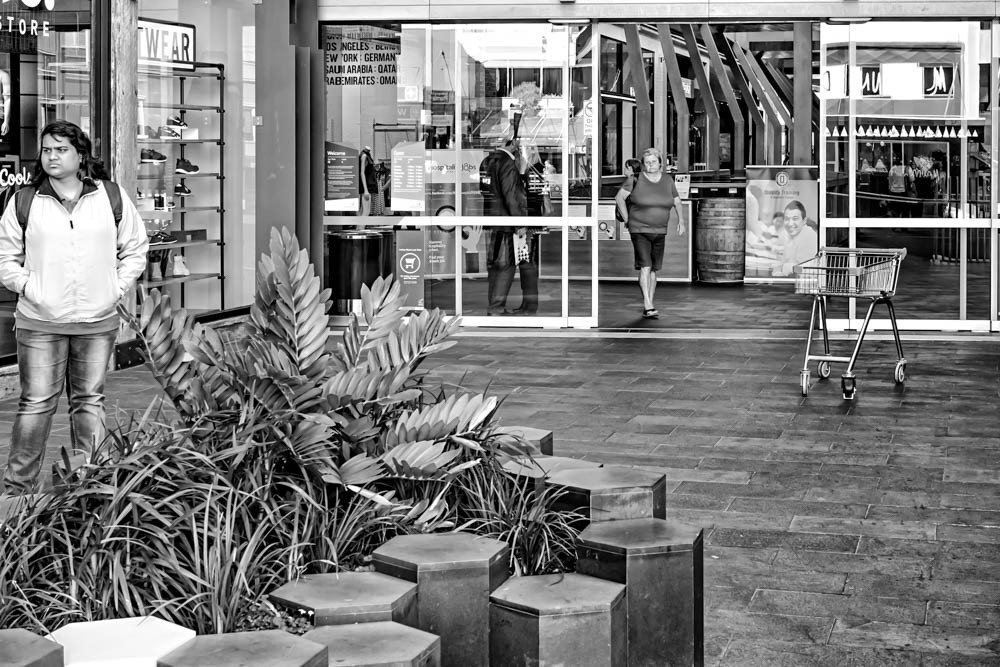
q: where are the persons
[614,147,686,319]
[624,159,642,180]
[888,153,915,233]
[772,199,817,275]
[478,123,527,316]
[0,117,150,505]
[513,138,548,316]
[769,211,785,234]
[861,144,887,172]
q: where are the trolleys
[792,247,908,400]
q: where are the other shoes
[642,309,659,317]
[487,308,512,316]
[513,308,535,315]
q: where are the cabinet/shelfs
[136,57,227,329]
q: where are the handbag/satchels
[616,173,639,222]
[903,166,917,201]
